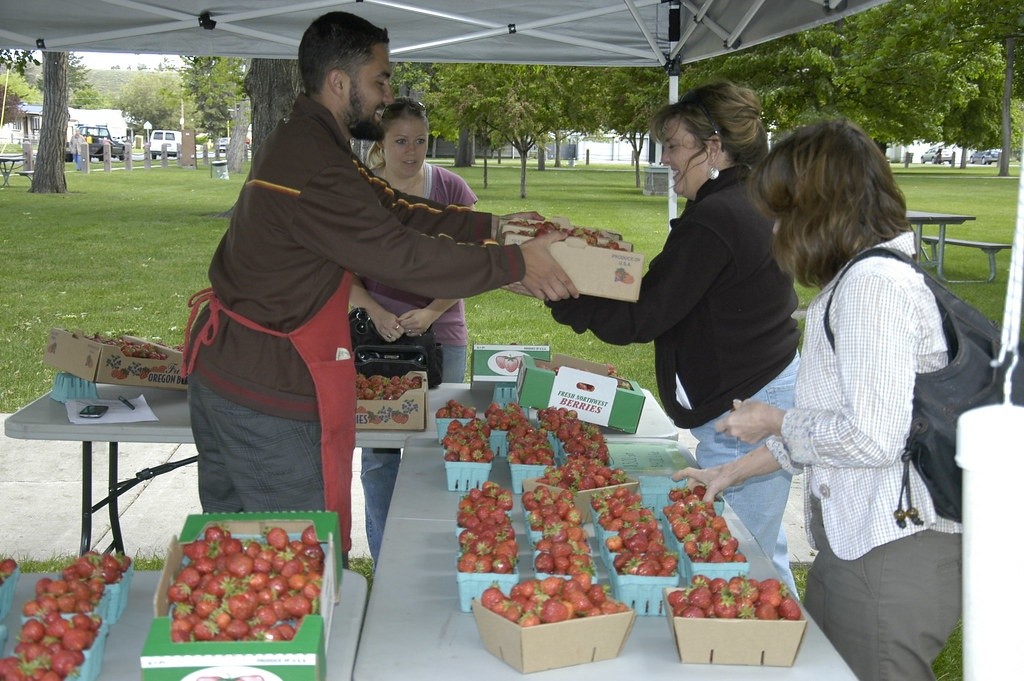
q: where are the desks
[352,435,859,681]
[5,380,680,557]
[0,568,368,681]
[0,156,33,189]
[904,210,1001,284]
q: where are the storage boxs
[520,505,543,548]
[0,511,344,681]
[607,560,680,616]
[49,373,100,404]
[354,371,428,432]
[519,476,639,523]
[515,353,647,435]
[491,382,517,408]
[587,502,644,570]
[531,541,593,562]
[435,406,615,493]
[661,588,807,666]
[455,494,520,614]
[43,326,189,390]
[470,597,637,675]
[637,474,687,520]
[532,555,599,584]
[664,487,726,517]
[469,215,646,392]
[661,512,750,586]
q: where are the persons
[672,119,962,681]
[178,15,580,517]
[70,128,86,172]
[500,78,804,605]
[86,132,93,144]
[347,96,479,565]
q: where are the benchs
[14,171,35,176]
[922,235,1012,250]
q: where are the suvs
[64,119,125,163]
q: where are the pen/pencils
[119,396,136,410]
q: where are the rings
[387,334,391,338]
[395,325,400,330]
[408,329,412,333]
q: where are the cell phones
[79,405,108,418]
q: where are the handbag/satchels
[823,246,1024,528]
[348,307,444,386]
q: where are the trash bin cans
[212,161,229,180]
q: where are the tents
[0,0,1024,237]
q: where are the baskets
[50,373,99,404]
[0,561,135,681]
[436,385,808,674]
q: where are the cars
[219,137,232,153]
[920,143,958,165]
[969,146,1002,165]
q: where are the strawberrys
[435,399,801,627]
[84,331,186,359]
[167,528,325,642]
[508,218,628,251]
[355,373,422,400]
[0,552,130,681]
[552,367,595,392]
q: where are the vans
[148,129,182,160]
